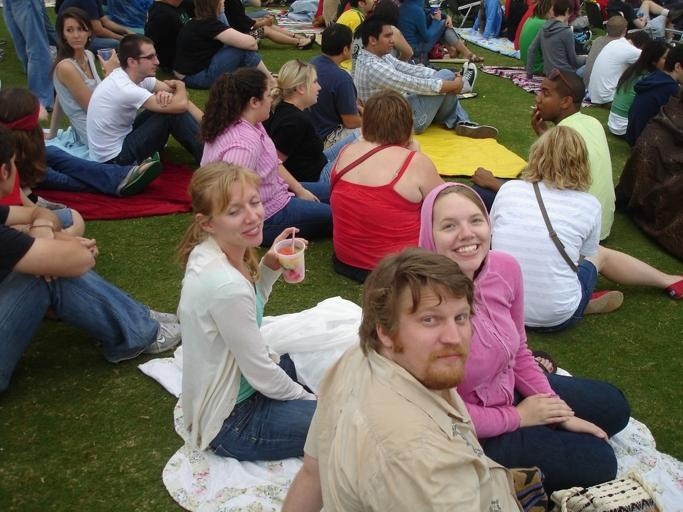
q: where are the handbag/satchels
[550,471,663,512]
[428,43,444,59]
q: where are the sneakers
[664,273,683,301]
[574,28,593,50]
[455,121,498,138]
[457,61,478,94]
[142,309,181,355]
[669,9,683,24]
[463,52,484,63]
[583,290,624,316]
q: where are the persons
[0,0,683,512]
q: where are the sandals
[532,349,557,375]
[292,34,315,51]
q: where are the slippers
[118,153,163,197]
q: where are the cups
[274,240,306,284]
[97,48,114,78]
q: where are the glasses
[296,57,308,77]
[577,0,585,9]
[132,52,157,60]
[545,67,576,103]
